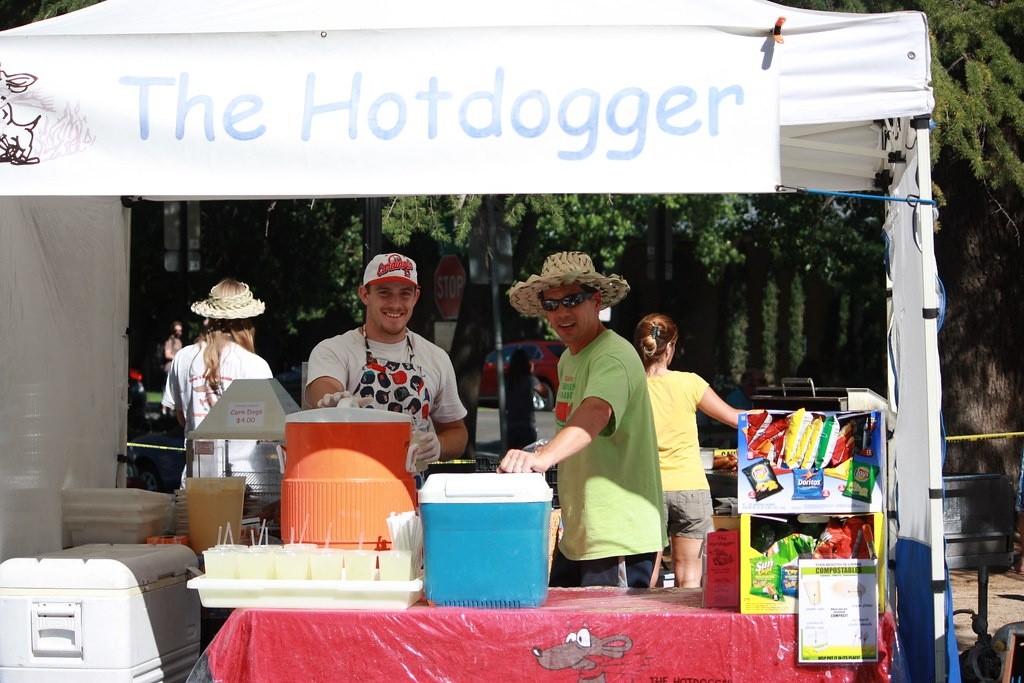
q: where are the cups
[412,419,429,472]
[201,543,413,581]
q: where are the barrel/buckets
[185,476,247,556]
[281,408,417,569]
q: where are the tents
[0,0,961,683]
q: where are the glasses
[541,293,593,312]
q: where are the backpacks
[153,336,175,369]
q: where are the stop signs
[431,255,467,319]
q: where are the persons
[304,254,468,484]
[504,350,549,450]
[632,314,767,588]
[497,251,669,590]
[163,321,183,374]
[160,279,284,516]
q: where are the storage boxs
[738,513,886,614]
[421,473,554,606]
[701,528,740,608]
[737,410,882,510]
[700,447,718,469]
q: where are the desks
[192,586,899,683]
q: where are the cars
[125,419,187,495]
[278,366,302,406]
[128,369,148,428]
[484,340,568,410]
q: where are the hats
[363,253,418,287]
[510,251,630,317]
[191,282,265,320]
